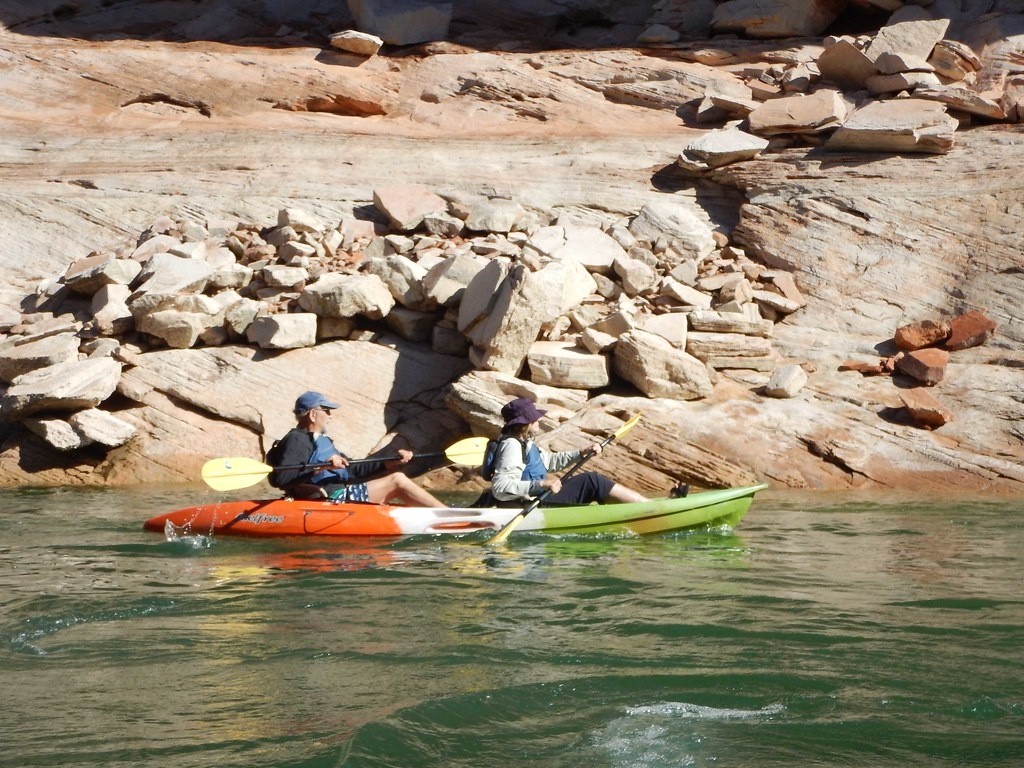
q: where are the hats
[293,391,340,414]
[501,398,548,435]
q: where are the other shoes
[668,482,689,499]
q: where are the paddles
[482,411,643,546]
[199,435,493,494]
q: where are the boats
[142,483,770,540]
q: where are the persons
[491,397,690,505]
[276,391,497,508]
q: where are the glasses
[314,408,330,415]
